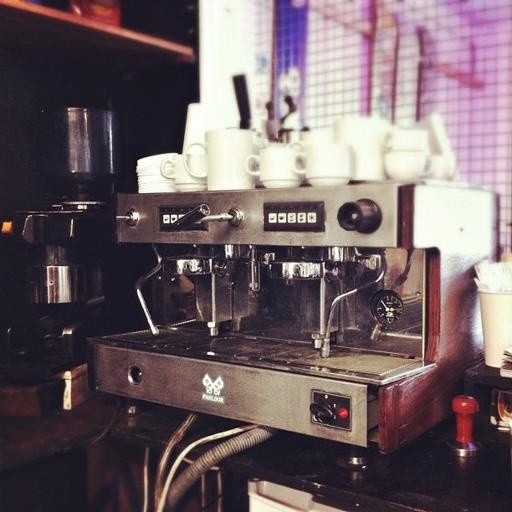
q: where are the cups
[161,99,428,189]
[477,289,512,370]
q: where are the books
[497,347,512,380]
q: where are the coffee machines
[91,185,500,449]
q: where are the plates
[136,152,178,195]
